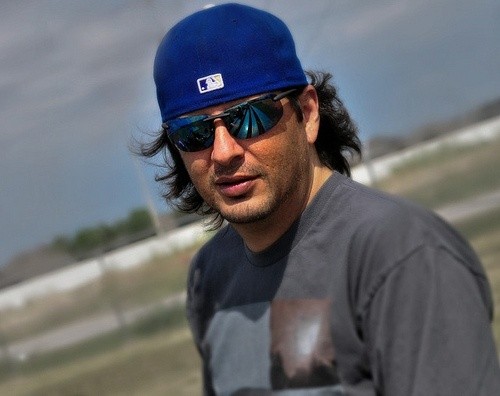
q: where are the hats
[154,4,309,121]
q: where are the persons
[154,0,500,396]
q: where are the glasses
[162,86,301,155]
